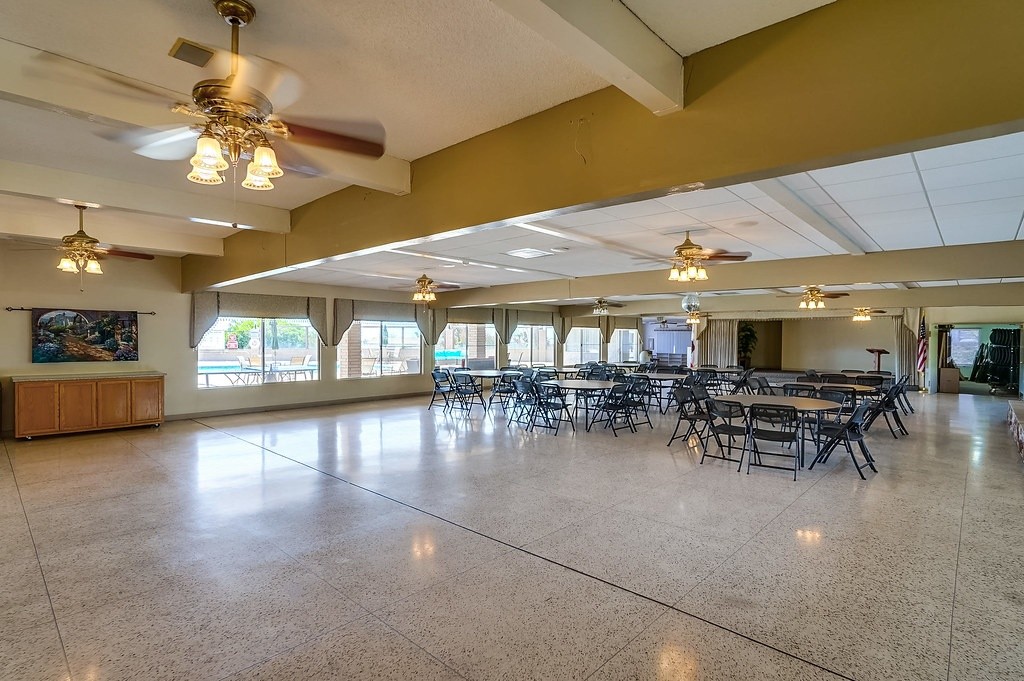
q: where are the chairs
[429,366,915,482]
[235,348,420,378]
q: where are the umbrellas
[269,318,279,373]
[383,324,388,371]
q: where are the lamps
[798,297,826,309]
[57,252,104,293]
[594,308,610,316]
[189,114,285,228]
[667,257,708,283]
[412,291,435,314]
[851,314,871,322]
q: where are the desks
[598,363,641,373]
[714,394,841,449]
[1008,399,1024,464]
[940,368,959,394]
[535,368,589,380]
[623,374,690,411]
[539,380,627,419]
[782,381,877,422]
[452,372,524,397]
[684,367,743,392]
[816,372,896,391]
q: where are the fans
[587,300,624,307]
[848,307,887,314]
[776,286,851,300]
[10,205,155,261]
[135,1,386,158]
[638,231,749,266]
[649,317,693,328]
[394,275,461,292]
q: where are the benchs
[434,350,464,367]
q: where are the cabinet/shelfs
[11,371,167,440]
[655,352,687,369]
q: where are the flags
[916,315,927,374]
